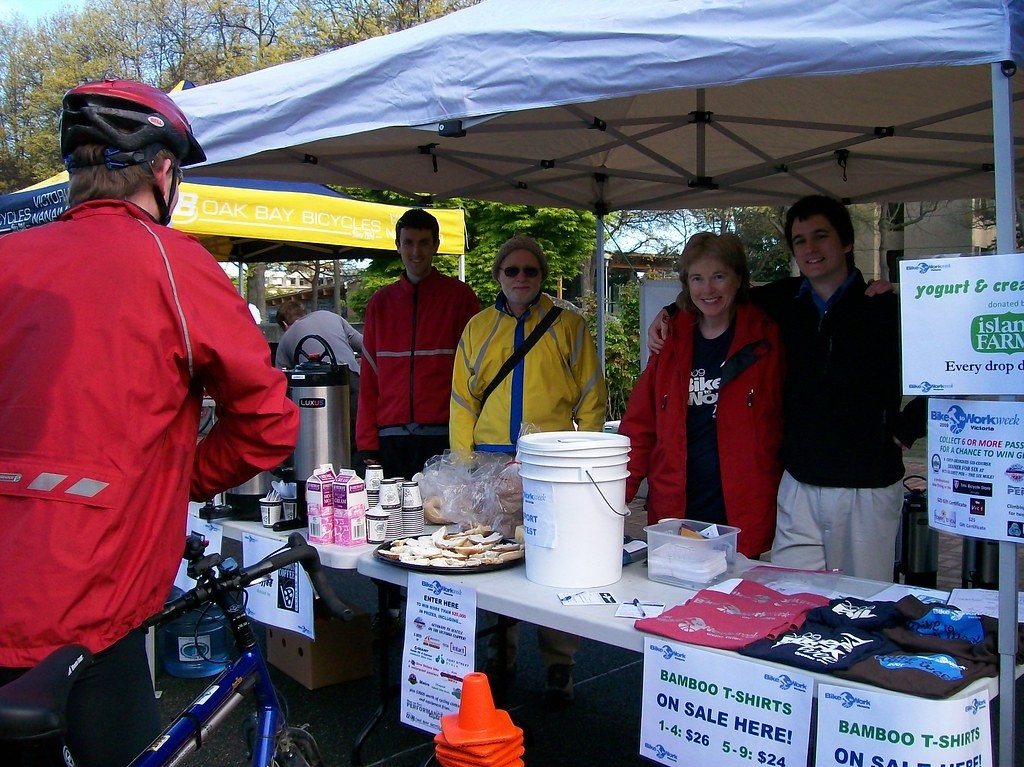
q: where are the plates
[375,534,524,574]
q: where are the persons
[272,300,364,473]
[449,233,608,696]
[612,230,895,565]
[648,193,969,585]
[355,207,484,638]
[2,83,302,767]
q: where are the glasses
[498,266,541,277]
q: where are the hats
[492,235,549,281]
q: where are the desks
[186,497,1024,767]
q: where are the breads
[379,525,524,566]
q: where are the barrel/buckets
[160,587,231,678]
[520,431,632,589]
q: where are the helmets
[59,79,207,167]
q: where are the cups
[259,498,282,528]
[364,463,425,542]
[283,499,298,522]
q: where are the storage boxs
[265,599,371,690]
[643,519,742,591]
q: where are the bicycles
[0,531,355,767]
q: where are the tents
[2,78,468,365]
[150,0,1023,767]
